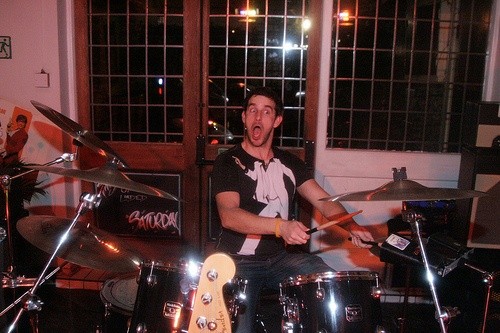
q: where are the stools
[223,285,283,333]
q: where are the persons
[2,115,29,166]
[211,86,375,333]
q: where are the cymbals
[16,215,150,274]
[19,159,184,203]
[317,178,492,203]
[30,100,122,161]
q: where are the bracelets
[274,217,283,239]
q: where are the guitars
[187,252,237,333]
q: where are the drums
[127,256,247,333]
[96,270,140,333]
[277,268,388,333]
